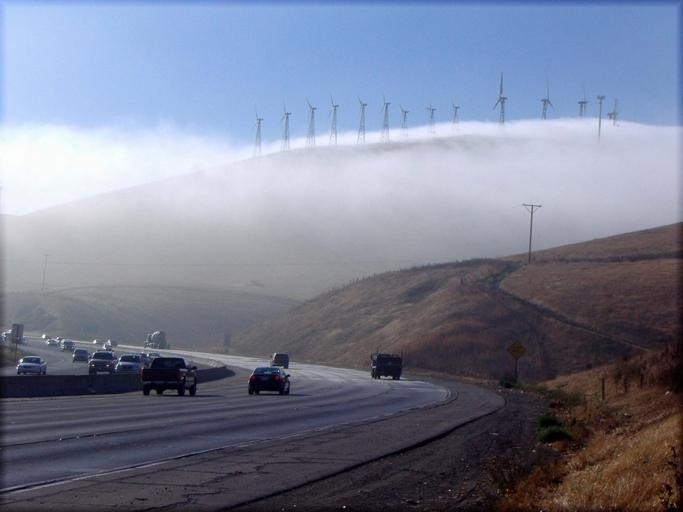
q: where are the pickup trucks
[140,356,198,397]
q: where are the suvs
[269,352,290,370]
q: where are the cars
[247,366,292,396]
[1,323,164,377]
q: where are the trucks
[368,351,403,381]
